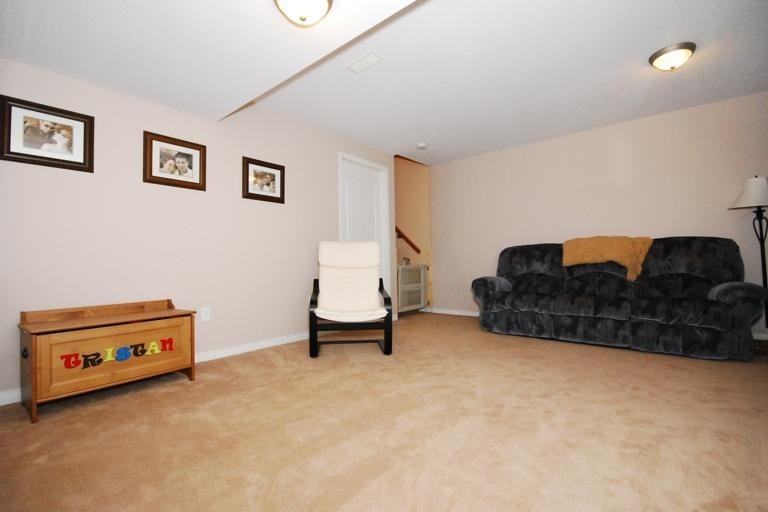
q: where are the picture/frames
[142,130,206,192]
[241,155,285,204]
[0,94,95,173]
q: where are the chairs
[308,239,397,357]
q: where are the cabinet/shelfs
[17,297,197,424]
[396,265,440,313]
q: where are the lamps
[272,0,334,27]
[725,174,767,357]
[648,41,696,70]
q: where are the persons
[254,169,271,194]
[160,153,176,173]
[24,117,57,151]
[37,124,74,157]
[263,171,276,194]
[170,152,194,178]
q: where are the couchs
[471,233,767,362]
